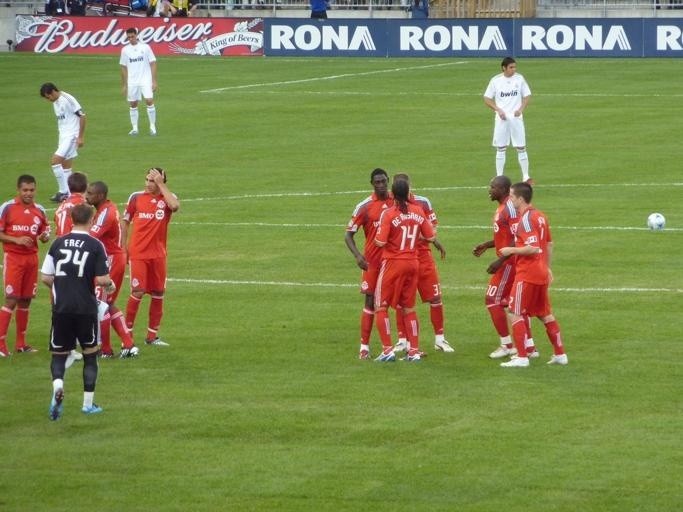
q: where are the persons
[0,174,51,361]
[308,1,328,18]
[410,0,429,19]
[39,82,85,202]
[53,172,88,239]
[116,27,161,137]
[38,201,112,423]
[121,167,178,348]
[496,181,569,368]
[483,56,533,186]
[343,167,447,361]
[391,171,455,356]
[470,175,539,360]
[373,180,435,363]
[85,180,139,361]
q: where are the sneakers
[0,346,13,358]
[49,385,65,421]
[490,344,539,368]
[50,191,69,201]
[149,127,157,136]
[128,129,139,136]
[98,342,139,359]
[527,178,534,186]
[547,353,569,366]
[81,403,103,413]
[15,344,39,354]
[144,337,170,346]
[434,339,454,352]
[359,342,428,362]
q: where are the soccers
[648,213,665,231]
